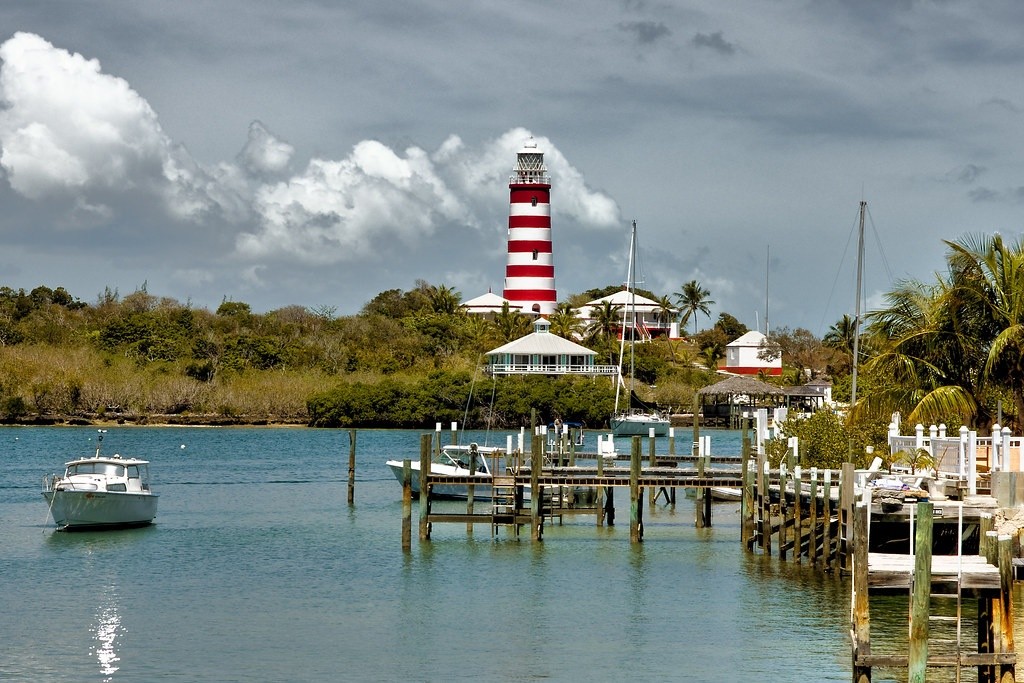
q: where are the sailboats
[607,219,674,438]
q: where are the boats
[546,422,586,453]
[39,435,160,527]
[385,457,571,503]
